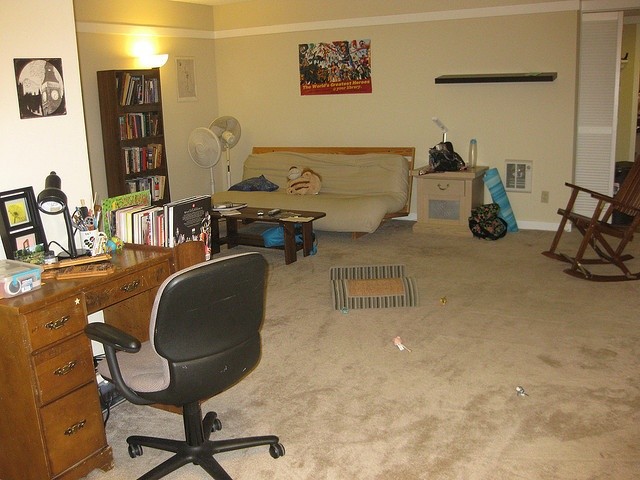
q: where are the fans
[208,116,242,189]
[188,127,222,196]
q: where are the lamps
[431,117,449,143]
[36,172,91,260]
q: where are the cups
[468,140,478,168]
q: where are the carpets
[330,263,418,311]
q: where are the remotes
[268,208,281,215]
[257,210,264,214]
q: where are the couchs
[212,146,415,240]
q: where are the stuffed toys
[285,165,310,196]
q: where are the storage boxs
[1,262,44,301]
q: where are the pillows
[228,175,279,192]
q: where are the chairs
[541,154,639,282]
[84,251,286,480]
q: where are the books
[100,188,214,261]
[114,72,160,106]
[123,175,167,201]
[117,111,162,141]
[120,143,164,174]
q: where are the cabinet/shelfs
[97,68,170,207]
[101,241,210,416]
[409,163,489,238]
[0,250,172,479]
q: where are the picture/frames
[0,187,50,260]
[175,56,197,103]
